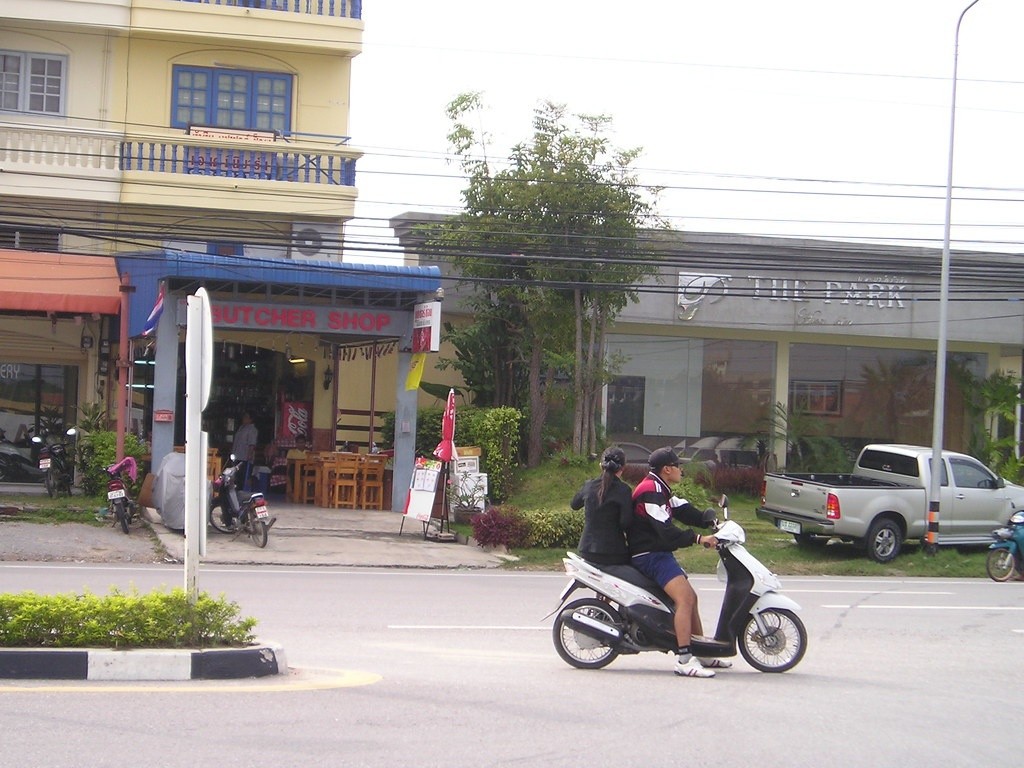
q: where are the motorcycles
[208,453,269,549]
[31,427,76,497]
[986,499,1024,582]
[100,462,138,534]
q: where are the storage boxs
[448,445,488,520]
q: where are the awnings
[0,248,121,314]
[115,250,442,340]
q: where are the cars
[607,441,692,484]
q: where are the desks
[294,458,382,508]
[140,455,222,498]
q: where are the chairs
[173,442,223,497]
[286,450,387,509]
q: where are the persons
[231,411,257,509]
[570,444,632,617]
[286,435,314,504]
[626,446,732,678]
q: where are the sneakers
[673,656,716,677]
[697,657,732,667]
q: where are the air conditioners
[292,218,340,260]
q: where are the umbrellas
[433,388,460,534]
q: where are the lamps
[322,365,333,390]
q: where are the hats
[648,446,689,469]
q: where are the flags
[142,284,165,338]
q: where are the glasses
[669,463,678,468]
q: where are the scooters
[535,494,811,671]
[0,424,47,483]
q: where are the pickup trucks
[755,443,1024,564]
